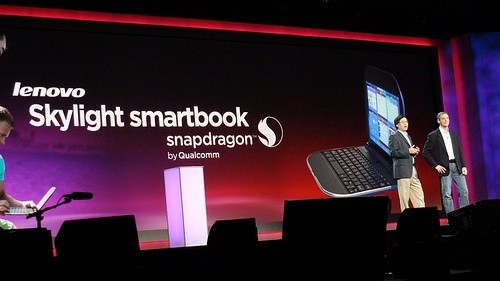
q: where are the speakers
[394,207,441,240]
[1,227,55,281]
[205,217,259,246]
[53,214,140,281]
[446,198,500,236]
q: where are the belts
[449,160,456,163]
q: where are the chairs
[0,194,500,281]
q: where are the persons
[388,116,425,213]
[0,106,36,230]
[421,111,469,213]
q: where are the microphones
[63,191,94,200]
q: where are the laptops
[306,64,406,198]
